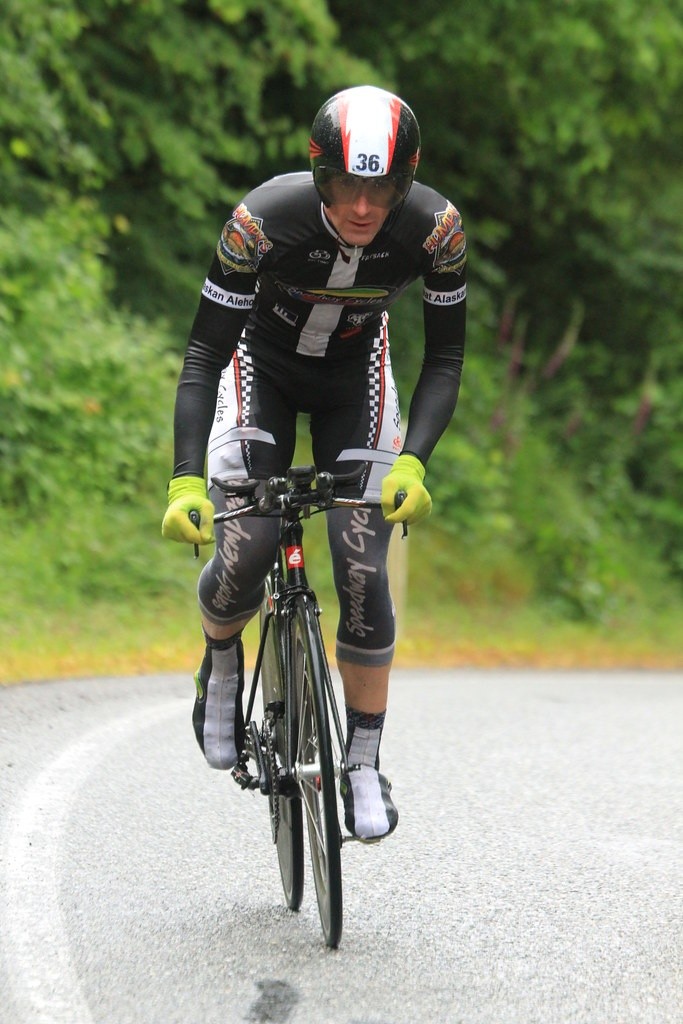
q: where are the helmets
[309,84,420,210]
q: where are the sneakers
[339,766,397,840]
[192,642,245,771]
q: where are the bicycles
[189,462,407,949]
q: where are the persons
[161,86,468,842]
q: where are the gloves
[161,475,216,545]
[380,456,432,529]
